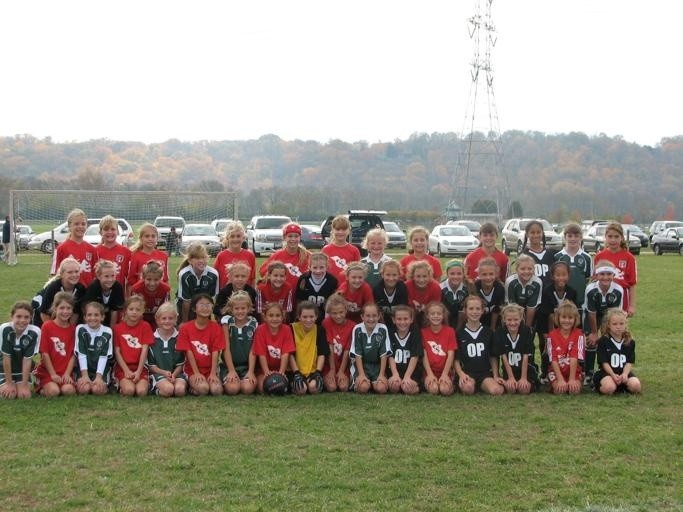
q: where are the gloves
[293,370,307,391]
[307,370,323,393]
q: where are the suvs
[321,210,388,254]
[501,218,561,254]
[247,214,294,256]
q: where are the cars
[445,220,483,238]
[0,215,245,257]
[381,221,408,248]
[427,225,479,255]
[300,225,322,250]
[555,218,646,255]
[650,227,682,255]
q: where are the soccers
[263,373,289,396]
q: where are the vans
[648,220,682,240]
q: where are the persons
[166,227,182,257]
[95,215,132,286]
[50,210,100,285]
[320,216,361,286]
[0,243,641,399]
[463,223,509,288]
[554,225,595,308]
[589,224,638,319]
[400,227,441,283]
[213,219,256,289]
[519,220,555,384]
[3,216,9,263]
[259,222,311,286]
[361,228,392,275]
[127,224,169,302]
